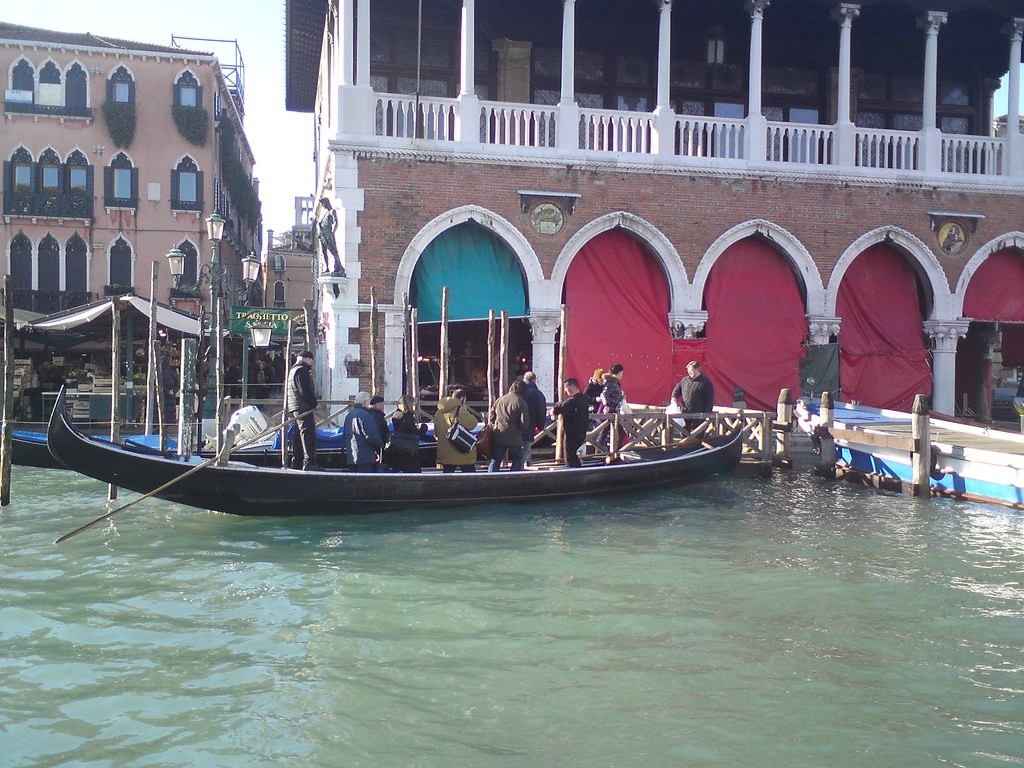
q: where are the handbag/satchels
[446,423,477,454]
[391,419,419,457]
[594,402,606,424]
[665,398,685,429]
[476,425,496,460]
[620,396,633,422]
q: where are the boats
[12,430,211,472]
[792,393,1024,511]
[44,385,746,515]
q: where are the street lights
[166,210,274,456]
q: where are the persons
[434,389,477,473]
[253,359,270,409]
[671,361,714,440]
[344,392,428,473]
[583,364,626,454]
[288,351,323,471]
[553,377,589,468]
[488,380,529,473]
[523,371,547,463]
[318,198,342,272]
[152,356,180,434]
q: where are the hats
[370,396,384,405]
[297,350,313,360]
